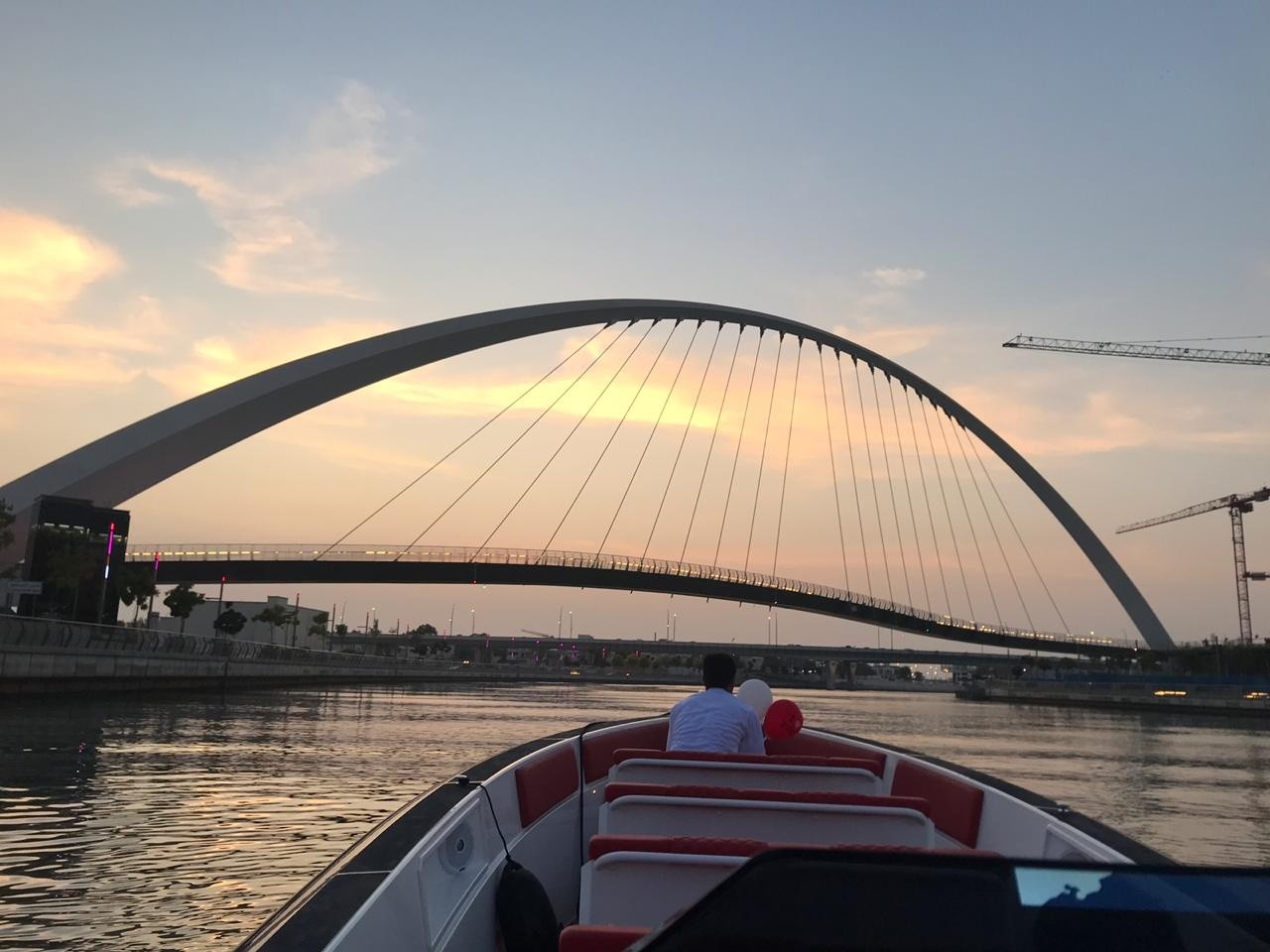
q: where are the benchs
[559,748,934,952]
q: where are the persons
[666,653,766,754]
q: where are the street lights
[569,610,574,640]
[767,614,772,646]
[665,609,670,642]
[371,607,375,622]
[449,602,455,635]
[557,606,564,639]
[672,613,677,642]
[470,607,476,635]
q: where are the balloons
[736,679,773,720]
[763,699,804,740]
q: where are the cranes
[1001,334,1270,367]
[1115,486,1270,646]
